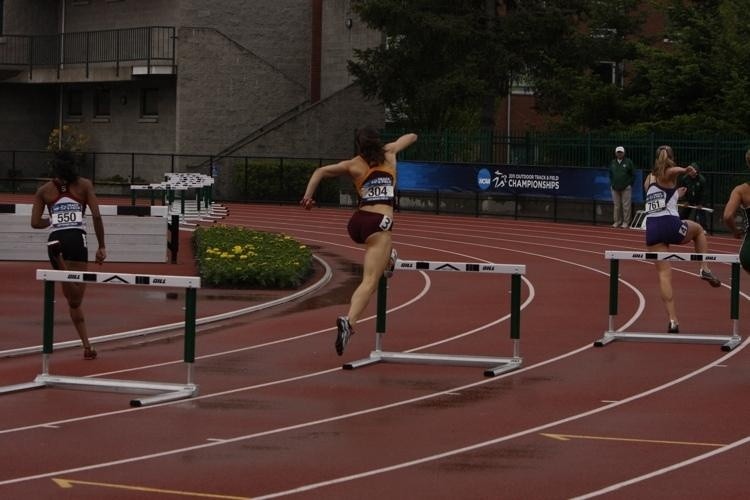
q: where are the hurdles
[341,250,527,378]
[0,202,179,264]
[593,250,740,352]
[0,269,201,406]
[128,171,215,218]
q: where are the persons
[299,124,418,357]
[723,150,749,277]
[644,145,721,334]
[608,146,636,229]
[31,150,106,361]
[680,163,707,231]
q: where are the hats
[689,162,699,168]
[615,146,625,154]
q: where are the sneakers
[383,248,399,279]
[620,221,629,229]
[611,221,621,228]
[334,315,354,357]
[699,267,721,288]
[46,239,62,256]
[667,319,681,333]
[82,343,97,362]
